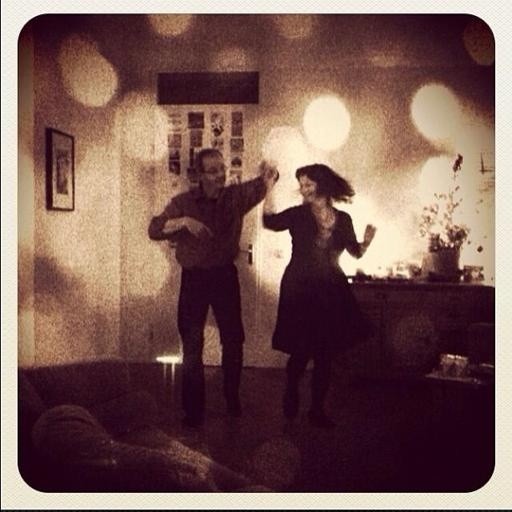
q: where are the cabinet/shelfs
[324,274,494,393]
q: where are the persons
[148,148,280,427]
[262,162,375,428]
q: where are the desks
[427,368,493,454]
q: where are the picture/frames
[43,126,79,212]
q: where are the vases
[422,248,461,275]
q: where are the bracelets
[361,244,367,251]
[182,215,187,226]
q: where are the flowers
[412,152,468,249]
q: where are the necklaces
[312,209,333,227]
